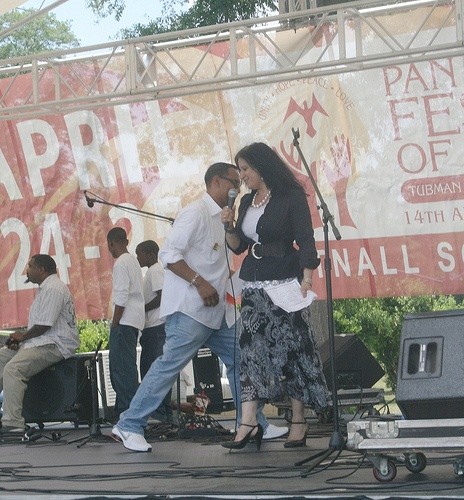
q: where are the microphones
[62,403,80,413]
[83,190,94,207]
[224,188,238,230]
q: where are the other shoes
[0,425,25,437]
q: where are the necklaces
[251,190,271,208]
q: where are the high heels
[283,418,309,449]
[221,423,263,451]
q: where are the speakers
[21,356,93,425]
[317,333,386,391]
[394,309,464,418]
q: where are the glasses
[219,175,242,189]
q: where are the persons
[0,254,80,433]
[107,227,145,426]
[111,162,289,453]
[221,142,333,453]
[135,240,172,421]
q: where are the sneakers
[110,423,152,453]
[261,424,288,439]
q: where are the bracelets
[190,272,200,287]
[301,278,313,285]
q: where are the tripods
[292,127,405,478]
[68,341,115,448]
